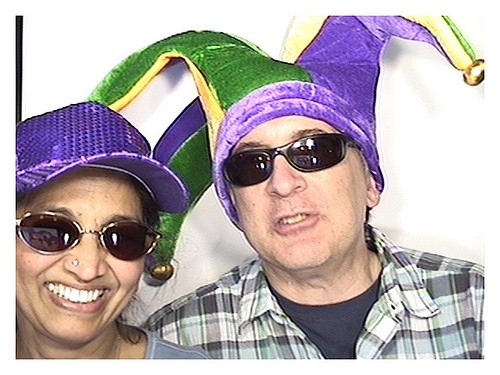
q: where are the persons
[16,101,215,359]
[82,16,486,360]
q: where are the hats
[84,12,486,228]
[16,102,191,213]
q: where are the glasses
[15,211,163,263]
[220,133,362,187]
